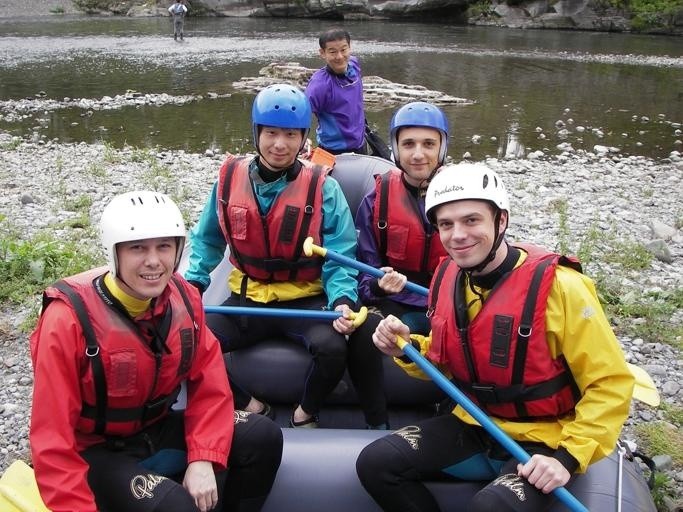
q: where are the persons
[354,162,634,512]
[183,82,359,430]
[349,100,448,432]
[29,189,284,511]
[305,27,370,155]
[163,1,188,41]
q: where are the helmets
[424,161,511,230]
[250,83,313,155]
[388,100,449,173]
[99,189,189,281]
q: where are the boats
[172,153,658,511]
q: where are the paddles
[0,458,54,512]
[302,236,661,409]
